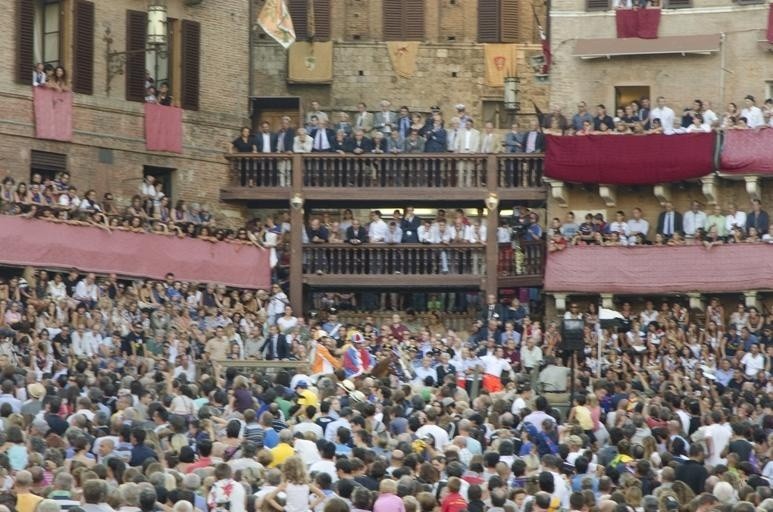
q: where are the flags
[257,0,296,50]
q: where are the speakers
[561,319,584,351]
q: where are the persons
[0,266,773,512]
[1,168,235,243]
[547,200,773,253]
[224,100,545,187]
[610,0,665,11]
[143,69,175,107]
[539,95,771,135]
[32,62,67,93]
[237,206,542,274]
